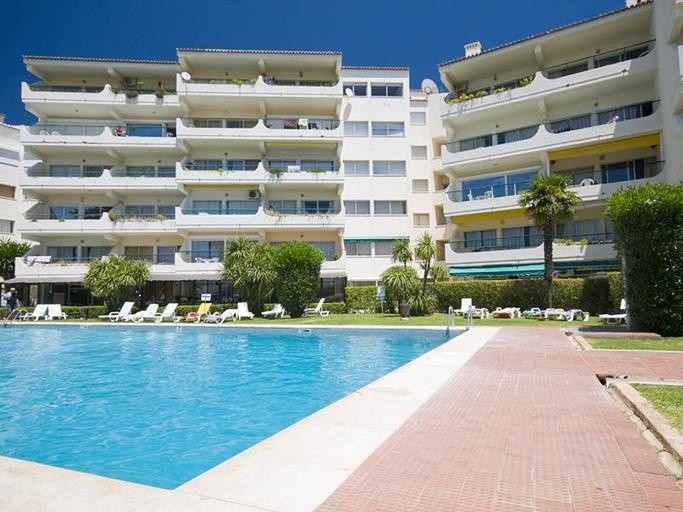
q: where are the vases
[399,303,411,316]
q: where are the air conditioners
[123,77,138,86]
[247,189,260,198]
[455,81,468,92]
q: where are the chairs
[97,301,255,323]
[581,178,594,186]
[599,312,627,325]
[17,304,68,320]
[454,306,589,321]
[303,297,330,318]
[260,303,286,318]
[484,191,494,199]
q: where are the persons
[1,286,20,320]
